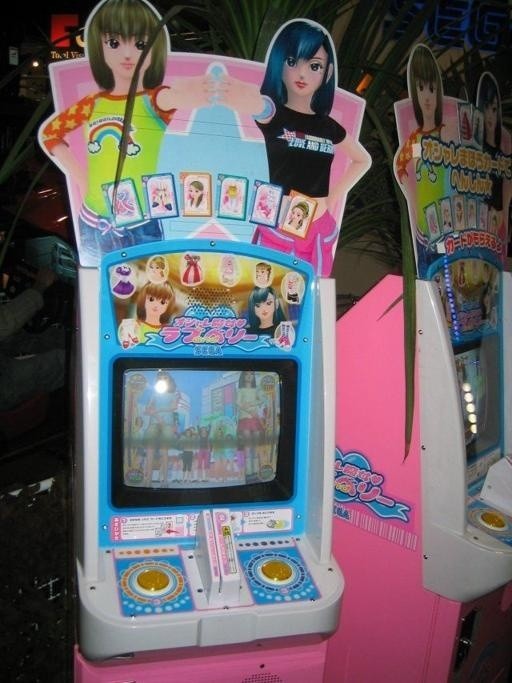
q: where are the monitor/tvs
[447,330,502,474]
[108,355,297,510]
